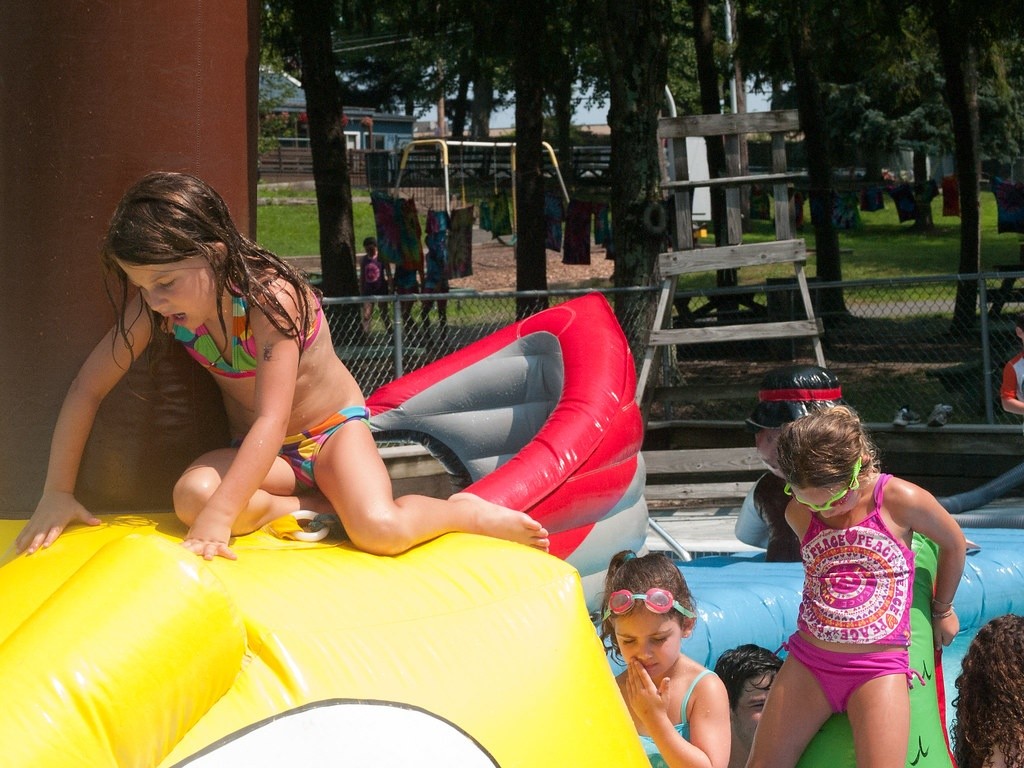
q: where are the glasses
[783,456,862,512]
[603,588,694,621]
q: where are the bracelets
[931,598,954,618]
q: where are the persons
[391,251,425,347]
[713,644,784,768]
[949,613,1024,768]
[746,408,967,768]
[735,364,847,562]
[1000,316,1024,415]
[422,236,450,346]
[599,551,731,768]
[360,237,394,334]
[16,170,550,561]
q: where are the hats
[744,364,849,428]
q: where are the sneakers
[927,404,948,425]
[892,404,922,425]
